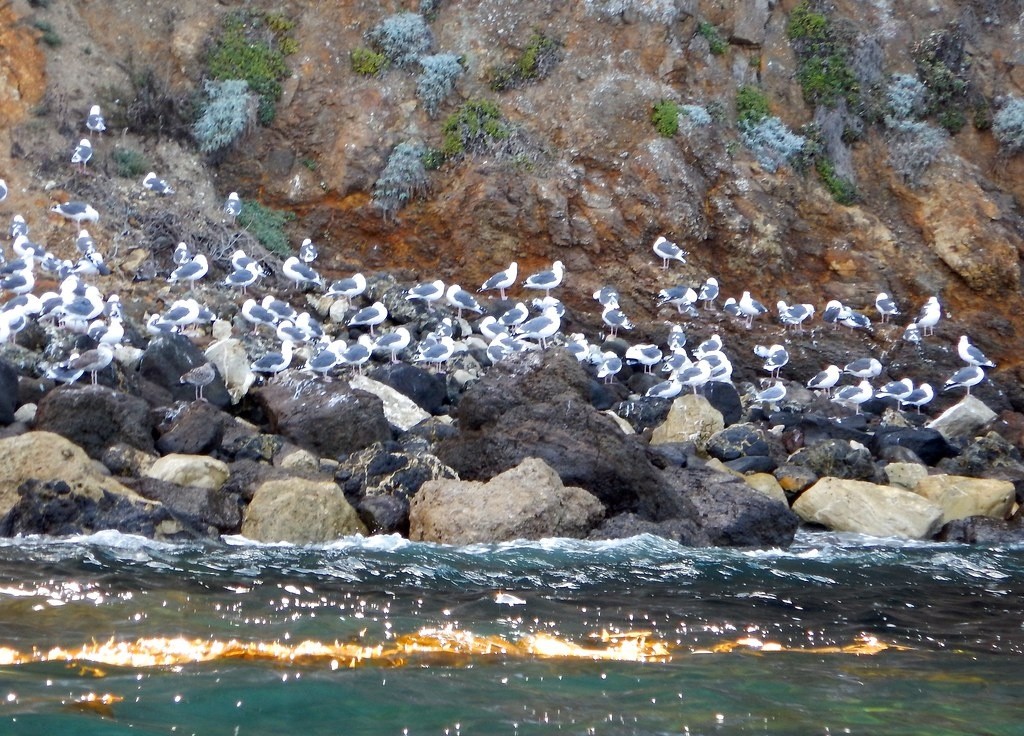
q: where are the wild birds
[48,199,100,237]
[85,104,107,140]
[143,172,176,197]
[0,214,996,416]
[0,179,8,201]
[223,192,242,225]
[71,139,93,174]
